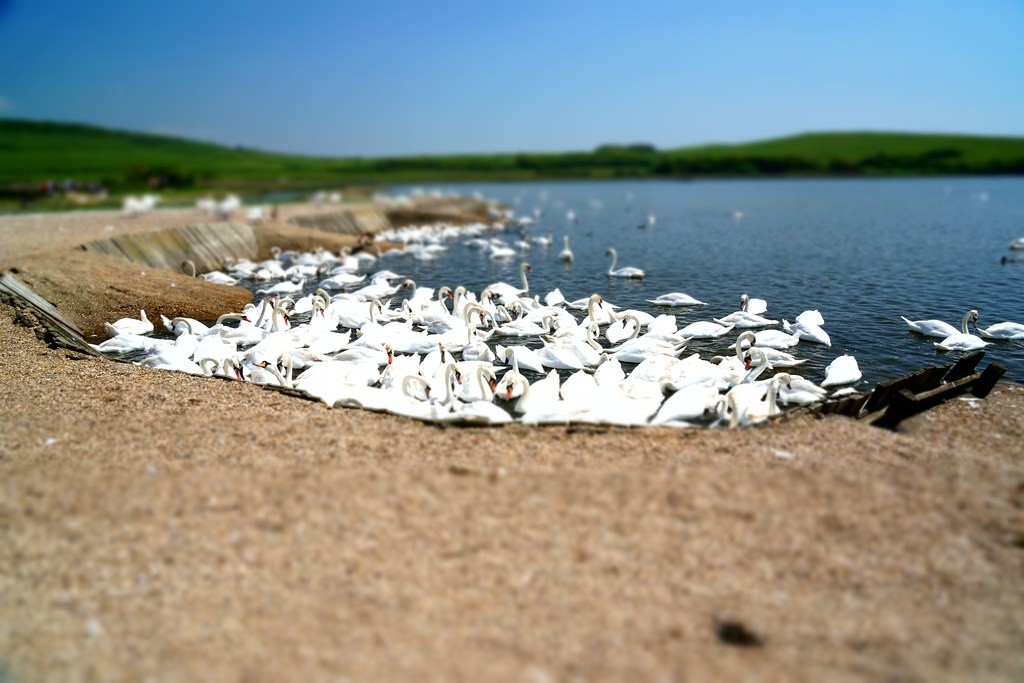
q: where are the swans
[86,181,864,433]
[901,309,1024,352]
